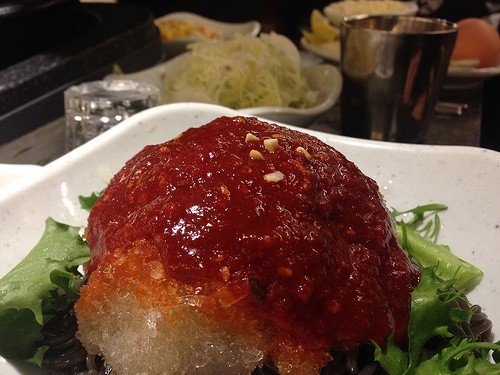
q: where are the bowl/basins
[325,0,418,28]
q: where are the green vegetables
[0,188,500,375]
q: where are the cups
[64,78,160,153]
[340,16,458,142]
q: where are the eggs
[449,18,500,68]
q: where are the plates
[155,13,259,42]
[0,100,500,375]
[106,52,342,127]
[302,34,500,81]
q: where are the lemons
[310,8,340,42]
